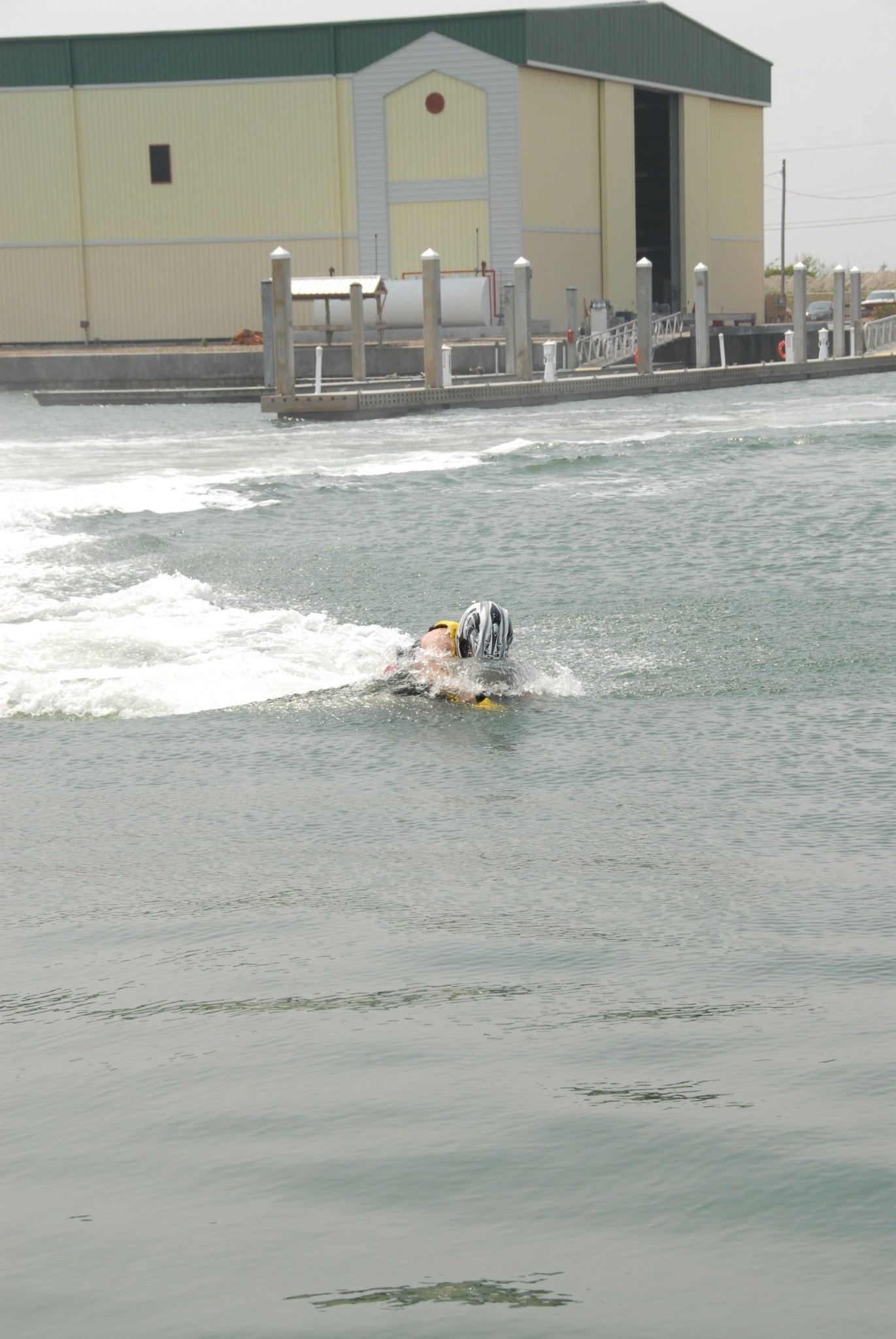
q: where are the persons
[786,306,792,324]
[398,600,513,705]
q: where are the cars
[860,290,896,319]
[806,300,834,321]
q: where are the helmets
[453,601,513,661]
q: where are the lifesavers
[778,340,785,359]
[633,348,638,365]
